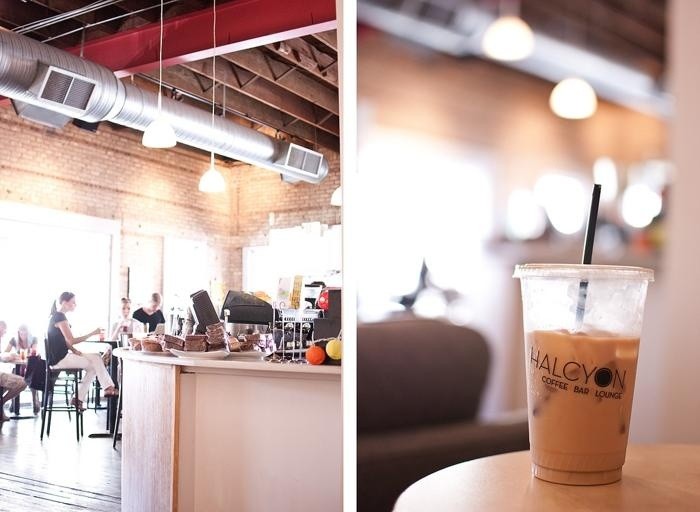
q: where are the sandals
[104,386,119,397]
[72,398,86,411]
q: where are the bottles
[99,328,106,341]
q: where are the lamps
[197,2,229,196]
[138,2,179,150]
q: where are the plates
[140,350,170,356]
[226,349,273,361]
[129,349,141,354]
[169,349,228,362]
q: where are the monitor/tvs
[189,290,220,335]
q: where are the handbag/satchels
[24,355,61,391]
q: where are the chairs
[39,336,85,443]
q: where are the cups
[512,261,653,489]
[122,320,131,332]
[29,347,36,356]
[20,348,27,362]
[132,322,144,338]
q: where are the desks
[111,346,129,448]
[84,337,123,439]
[7,356,38,424]
[392,441,700,512]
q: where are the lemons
[326,340,342,360]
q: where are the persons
[0,291,166,422]
